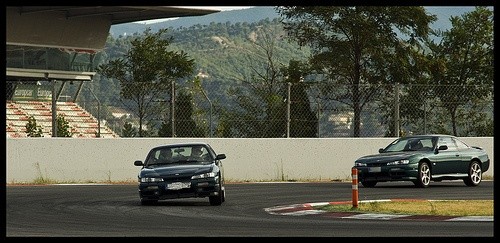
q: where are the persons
[188,146,204,162]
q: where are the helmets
[191,146,204,157]
[159,149,172,160]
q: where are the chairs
[158,148,174,163]
[410,140,423,151]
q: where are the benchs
[6,101,121,139]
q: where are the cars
[132,141,228,205]
[353,133,490,188]
